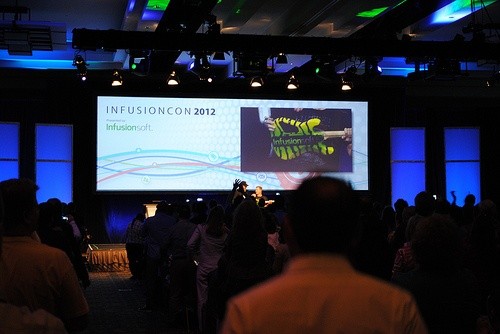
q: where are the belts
[173,256,188,260]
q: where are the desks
[143,204,158,219]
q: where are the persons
[0,178,89,334]
[349,188,500,334]
[257,108,352,172]
[31,193,90,289]
[126,181,293,334]
[217,176,426,334]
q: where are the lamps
[72,55,89,76]
[188,52,216,82]
[129,48,158,80]
[167,71,180,85]
[286,74,300,89]
[111,70,123,86]
[250,75,264,87]
[340,57,382,89]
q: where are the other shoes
[135,304,153,313]
[83,279,90,287]
[167,320,186,329]
[160,300,168,308]
[134,269,141,274]
